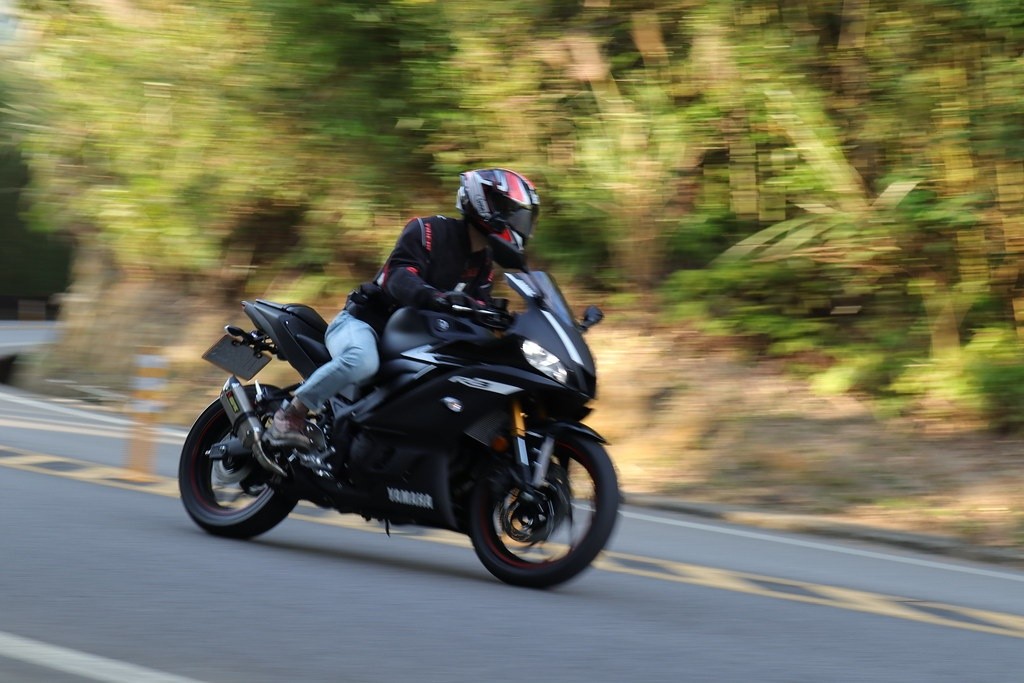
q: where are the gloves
[442,290,479,310]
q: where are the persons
[262,168,540,455]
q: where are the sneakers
[261,397,311,450]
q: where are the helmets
[454,166,540,253]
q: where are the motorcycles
[176,266,620,588]
